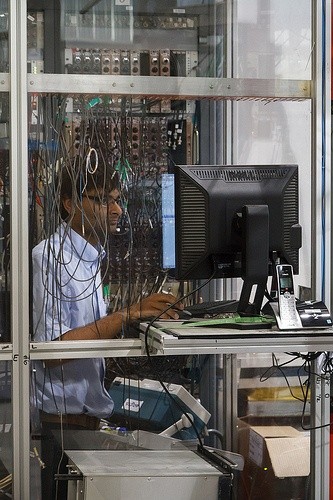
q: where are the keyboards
[183,299,239,315]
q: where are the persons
[29,159,185,500]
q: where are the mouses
[168,305,192,320]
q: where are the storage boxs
[248,425,311,479]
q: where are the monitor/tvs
[174,162,303,330]
[157,172,176,281]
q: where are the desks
[131,309,332,500]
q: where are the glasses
[83,192,121,205]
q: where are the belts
[39,410,100,428]
